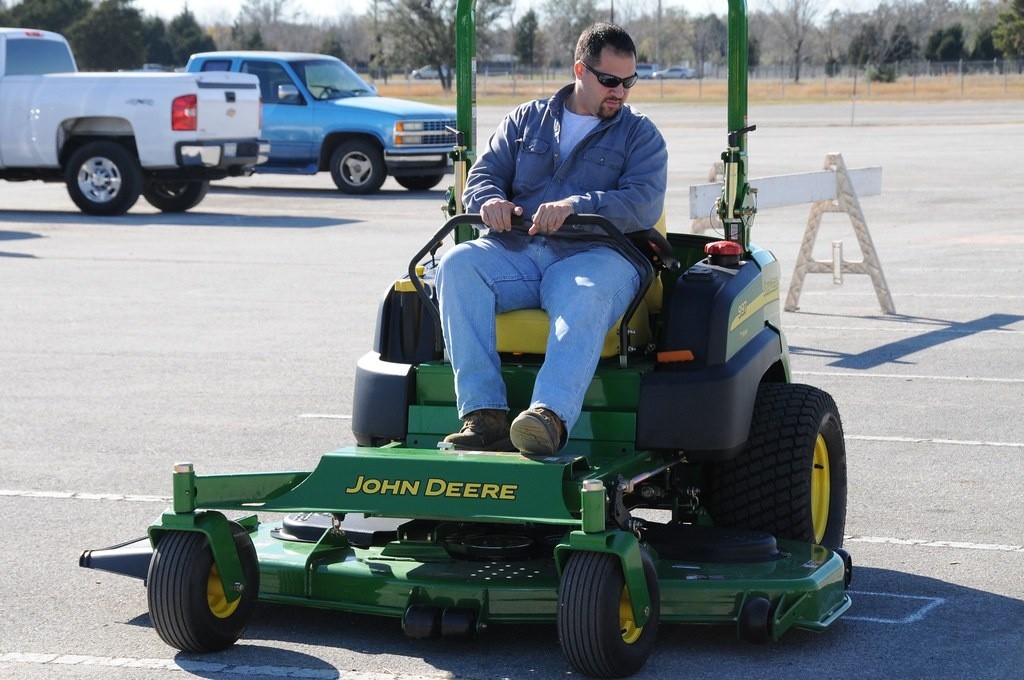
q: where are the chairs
[494,207,665,360]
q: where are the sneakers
[444,409,514,451]
[510,407,566,457]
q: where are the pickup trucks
[182,54,458,197]
[0,26,272,216]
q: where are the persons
[434,22,668,462]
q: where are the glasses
[580,60,638,89]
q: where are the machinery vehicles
[81,0,851,678]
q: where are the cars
[411,64,454,79]
[636,64,698,79]
